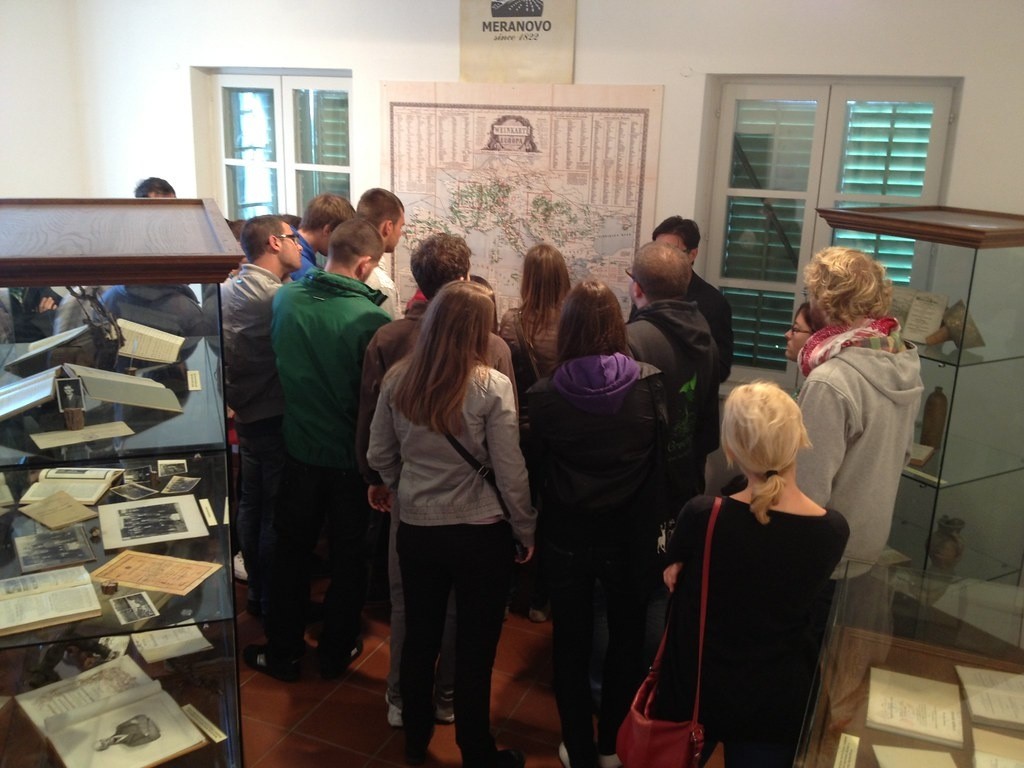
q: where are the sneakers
[243,645,302,682]
[385,688,406,726]
[318,638,363,679]
[432,691,457,723]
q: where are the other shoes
[558,741,624,768]
[405,728,434,764]
[461,750,526,768]
[502,603,516,621]
[529,601,551,622]
[247,598,262,616]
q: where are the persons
[93,713,161,752]
[0,178,922,768]
[63,384,83,409]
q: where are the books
[18,491,99,532]
[107,318,187,363]
[5,324,90,369]
[14,655,208,768]
[63,363,183,412]
[20,468,125,505]
[0,565,102,638]
[0,363,62,423]
[130,619,214,664]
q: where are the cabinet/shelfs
[817,207,1024,659]
[0,200,243,768]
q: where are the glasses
[791,326,811,333]
[625,267,645,295]
[266,234,298,246]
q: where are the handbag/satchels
[488,520,544,576]
[616,671,705,768]
[630,380,674,603]
